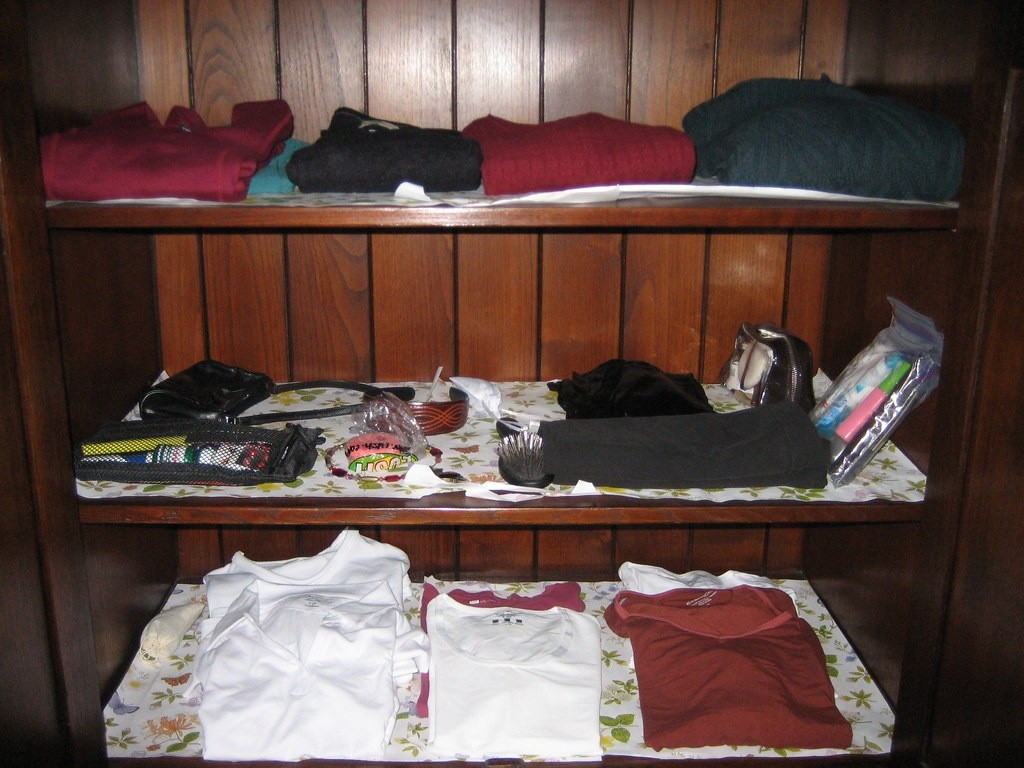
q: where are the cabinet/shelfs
[0,0,1024,768]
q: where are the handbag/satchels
[720,320,816,415]
[139,358,385,426]
